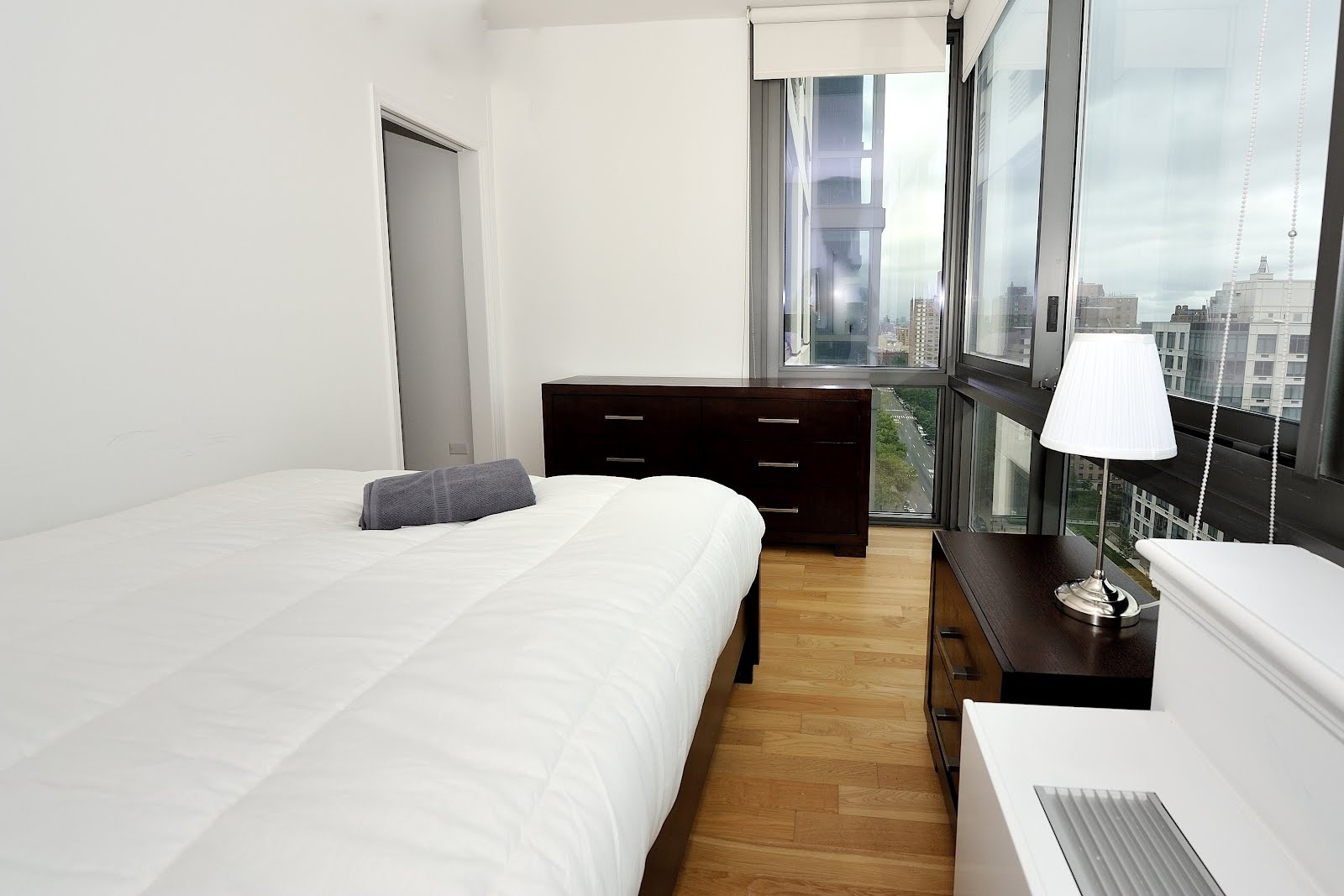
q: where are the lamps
[1039,333,1178,626]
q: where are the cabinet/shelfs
[924,529,1159,840]
[542,376,872,558]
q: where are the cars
[932,450,935,455]
[894,391,909,411]
[913,420,932,445]
[903,500,915,512]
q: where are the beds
[0,469,765,896]
